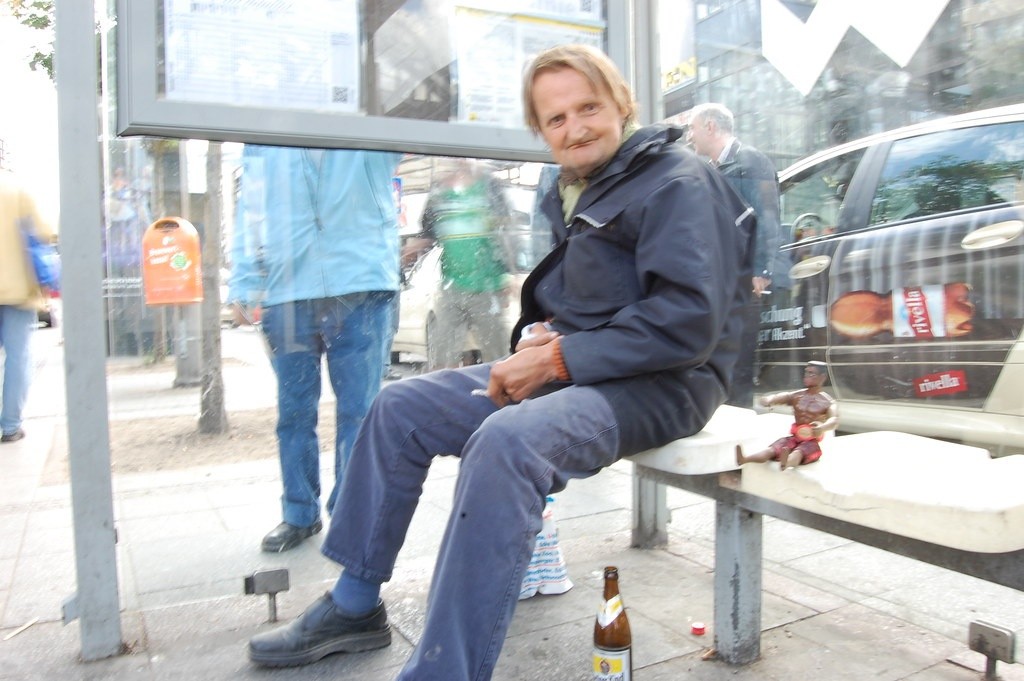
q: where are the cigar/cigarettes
[752,290,772,294]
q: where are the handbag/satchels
[27,235,62,297]
[517,496,574,600]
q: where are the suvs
[753,102,1024,456]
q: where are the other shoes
[1,428,24,441]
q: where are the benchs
[602,455,1024,669]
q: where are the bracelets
[554,341,570,380]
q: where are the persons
[736,360,838,471]
[0,168,56,442]
[402,155,520,372]
[230,136,404,550]
[247,45,756,681]
[685,104,792,410]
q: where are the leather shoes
[246,590,391,667]
[261,518,322,553]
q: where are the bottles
[592,566,632,680]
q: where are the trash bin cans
[141,216,205,306]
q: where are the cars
[393,240,541,369]
